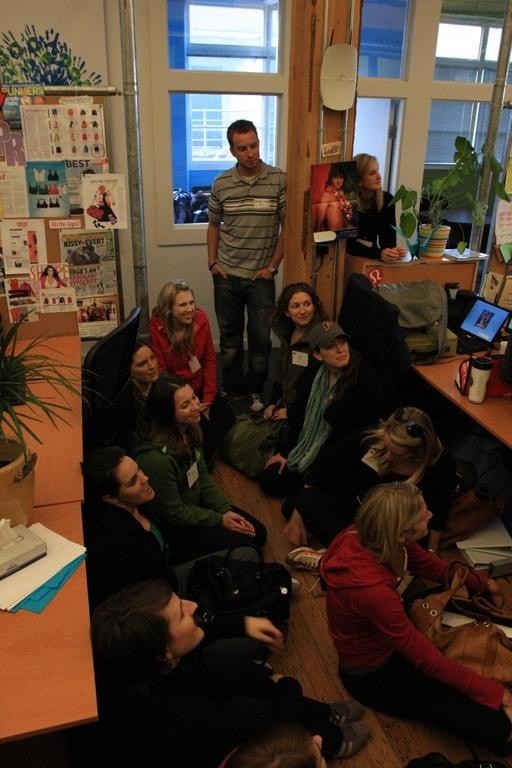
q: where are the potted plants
[0,301,99,531]
[384,134,512,260]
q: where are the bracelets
[208,262,217,270]
[267,265,278,275]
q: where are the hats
[306,319,350,349]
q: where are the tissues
[0,518,48,580]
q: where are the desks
[412,348,512,467]
[338,243,489,292]
[2,314,87,501]
[1,509,100,755]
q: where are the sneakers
[324,699,364,723]
[286,544,328,574]
[218,388,245,405]
[248,389,266,413]
[335,721,372,762]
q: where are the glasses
[392,407,429,442]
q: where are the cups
[469,356,491,403]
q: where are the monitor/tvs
[452,295,512,355]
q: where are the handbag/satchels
[368,278,454,366]
[417,561,512,684]
[184,542,295,644]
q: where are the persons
[207,119,288,418]
[83,281,512,768]
[315,165,348,231]
[346,153,406,263]
